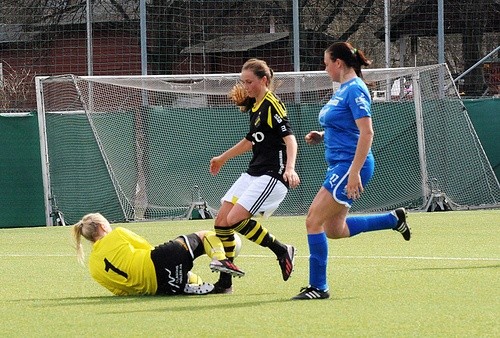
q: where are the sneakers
[184,282,214,295]
[389,208,412,241]
[209,258,245,278]
[276,244,297,281]
[291,286,330,301]
[213,283,232,293]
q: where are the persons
[290,42,413,300]
[210,58,299,293]
[71,213,245,295]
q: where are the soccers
[235,233,242,258]
